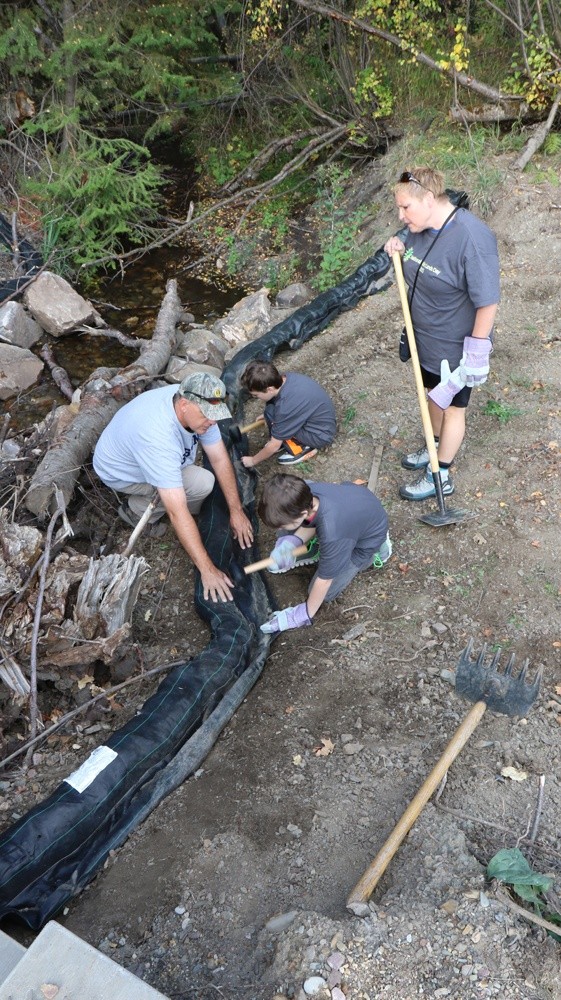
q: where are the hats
[178,372,233,421]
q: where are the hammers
[229,420,266,442]
[228,545,309,583]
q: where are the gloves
[266,534,303,571]
[260,602,313,634]
[459,336,494,388]
[427,359,466,409]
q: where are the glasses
[400,172,435,199]
[184,391,230,405]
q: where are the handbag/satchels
[399,327,411,362]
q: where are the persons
[385,167,501,499]
[256,474,392,633]
[241,359,337,468]
[94,372,254,603]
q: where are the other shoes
[118,500,168,537]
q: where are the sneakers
[265,441,285,452]
[267,534,321,574]
[399,463,454,500]
[276,445,318,465]
[400,442,439,470]
[373,530,393,567]
